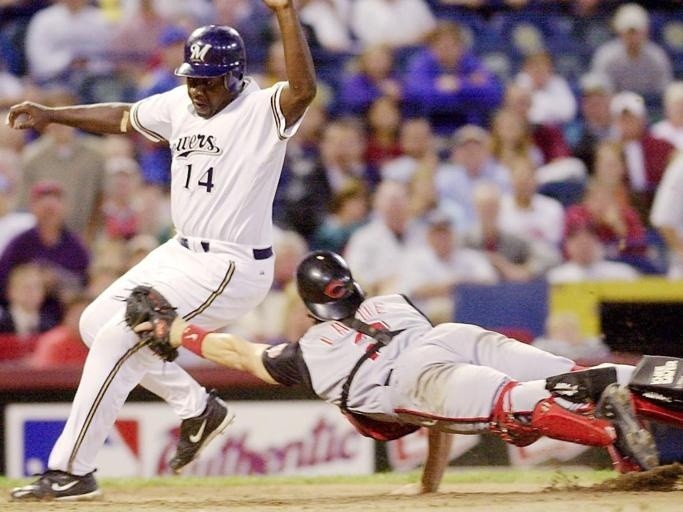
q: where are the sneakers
[170,387,235,472]
[11,471,100,503]
[592,384,660,470]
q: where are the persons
[0,0,683,333]
[3,3,316,503]
[122,248,681,473]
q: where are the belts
[178,237,274,260]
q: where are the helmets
[295,251,363,320]
[175,25,247,92]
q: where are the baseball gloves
[125,285,183,362]
